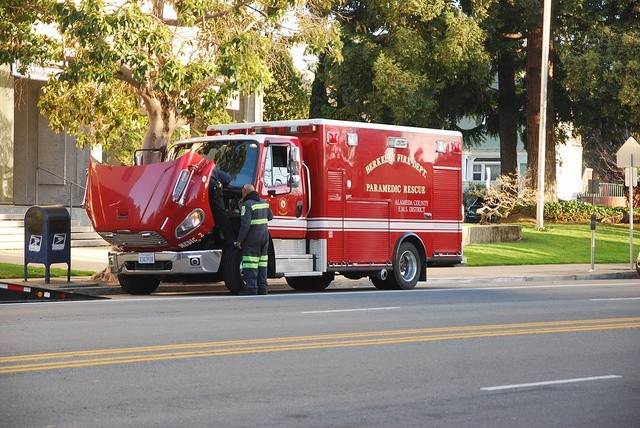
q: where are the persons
[235,184,273,296]
[209,165,233,246]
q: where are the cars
[466,195,499,221]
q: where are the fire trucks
[81,118,468,292]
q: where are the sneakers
[239,285,258,295]
[258,288,268,295]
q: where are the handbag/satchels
[215,181,223,197]
